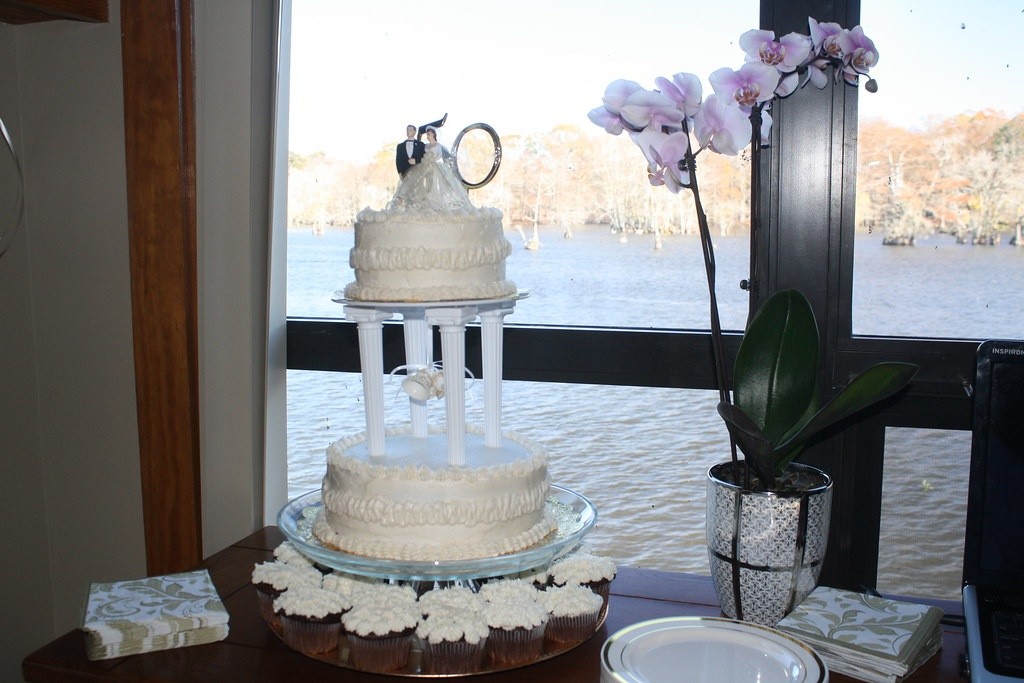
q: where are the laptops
[959,336,1024,683]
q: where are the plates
[276,481,599,583]
[600,615,829,683]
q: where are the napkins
[775,586,945,683]
[78,569,231,662]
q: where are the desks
[21,525,972,683]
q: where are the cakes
[309,418,559,562]
[344,126,519,303]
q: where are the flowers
[587,21,912,485]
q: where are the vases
[708,459,836,624]
